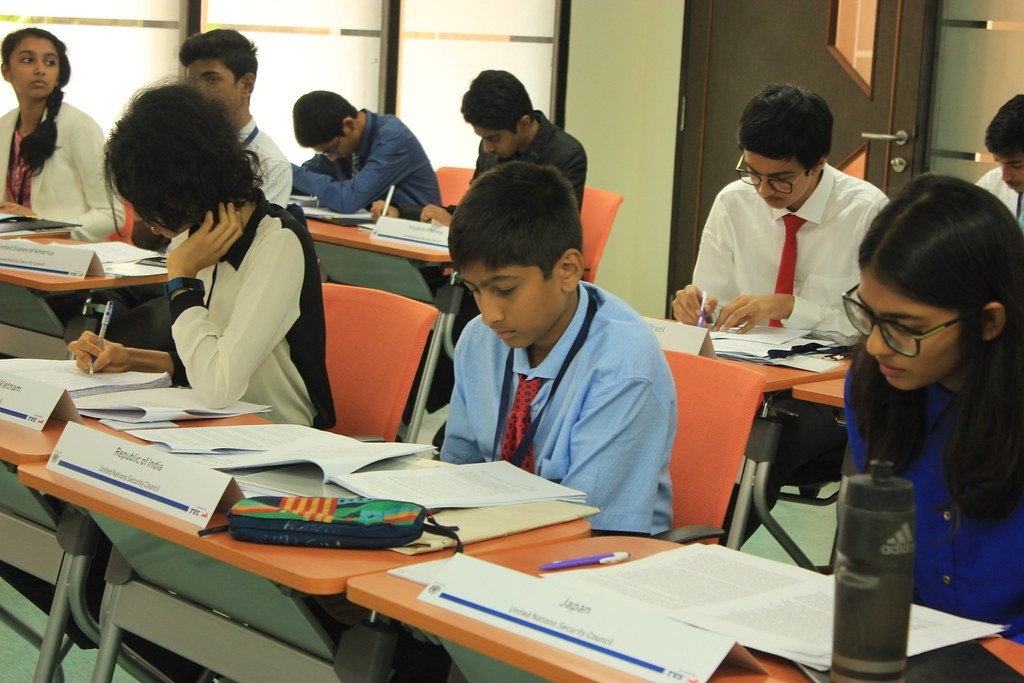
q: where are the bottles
[833,461,917,683]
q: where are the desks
[0,416,1024,683]
[307,218,462,361]
[662,317,846,572]
[0,232,168,360]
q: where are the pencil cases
[230,495,425,551]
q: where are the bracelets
[164,277,205,301]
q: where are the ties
[500,374,542,474]
[768,214,807,328]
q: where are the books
[0,357,273,422]
[290,194,376,231]
[675,319,843,373]
[540,540,1009,672]
[0,212,171,278]
[124,424,602,556]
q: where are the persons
[974,94,1024,234]
[67,80,336,429]
[841,176,1024,647]
[436,161,671,538]
[672,82,889,549]
[178,27,292,210]
[0,27,127,240]
[371,69,587,451]
[290,90,443,223]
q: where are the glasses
[842,283,980,357]
[314,135,342,159]
[735,154,819,194]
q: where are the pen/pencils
[90,299,115,373]
[536,552,632,571]
[697,287,710,327]
[379,183,398,217]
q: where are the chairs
[433,166,475,206]
[582,184,624,285]
[321,282,438,443]
[662,352,765,545]
